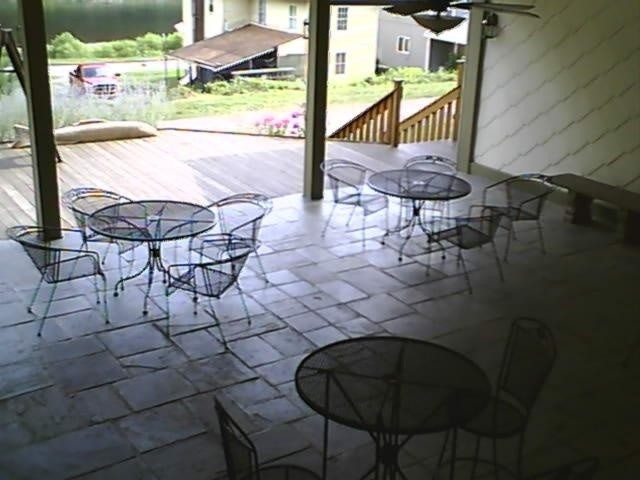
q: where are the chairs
[523,456,600,480]
[188,192,274,299]
[213,395,322,480]
[397,155,459,241]
[426,205,505,295]
[5,226,109,337]
[165,238,261,350]
[63,188,166,290]
[479,173,559,262]
[432,317,557,480]
[319,159,389,248]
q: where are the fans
[328,0,540,20]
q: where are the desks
[86,200,219,315]
[366,169,472,262]
[294,336,492,480]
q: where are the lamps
[481,13,505,40]
[412,0,466,33]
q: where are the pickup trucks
[67,60,122,102]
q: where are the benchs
[545,173,640,248]
